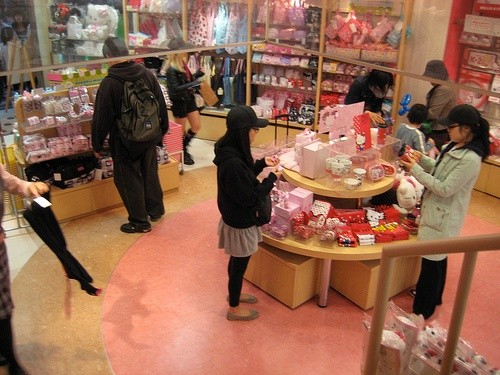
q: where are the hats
[438,104,481,126]
[226,105,268,128]
[422,60,449,80]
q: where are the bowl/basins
[344,177,358,190]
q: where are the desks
[245,162,421,308]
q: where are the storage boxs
[243,242,322,310]
[0,144,24,213]
[162,121,184,161]
[330,255,422,312]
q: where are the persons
[423,60,458,144]
[394,103,436,156]
[0,9,43,94]
[343,69,395,124]
[92,36,169,234]
[165,37,210,166]
[0,161,50,375]
[213,105,284,321]
[396,104,489,319]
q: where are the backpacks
[106,74,162,151]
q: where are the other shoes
[227,294,257,303]
[227,308,259,320]
[184,148,194,165]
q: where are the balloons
[398,94,411,116]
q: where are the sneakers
[408,288,416,297]
[150,209,165,221]
[120,222,151,233]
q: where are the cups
[371,128,378,147]
[378,124,388,144]
[340,159,352,176]
[335,154,347,163]
[325,157,338,174]
[331,162,344,181]
[353,167,366,186]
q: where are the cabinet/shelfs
[15,85,180,224]
[123,0,414,150]
[443,0,499,198]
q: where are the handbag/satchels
[255,194,272,226]
